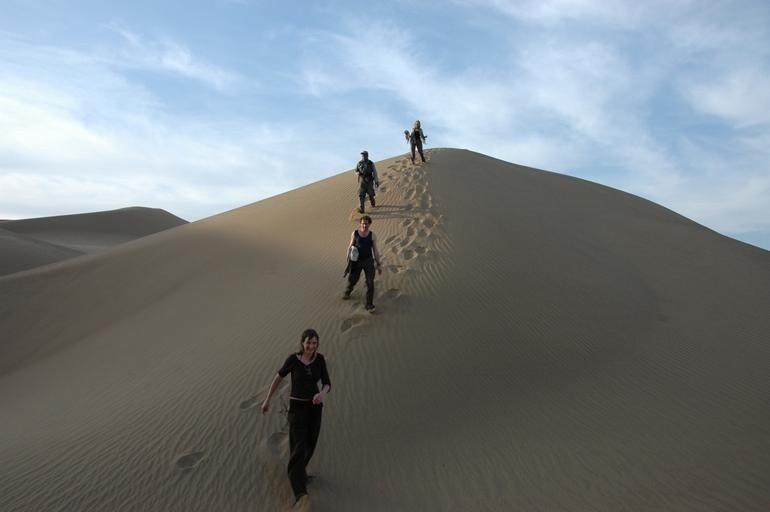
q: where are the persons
[260,328,334,505]
[404,120,428,164]
[354,151,379,214]
[343,216,383,313]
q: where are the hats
[350,246,359,262]
[360,151,368,155]
